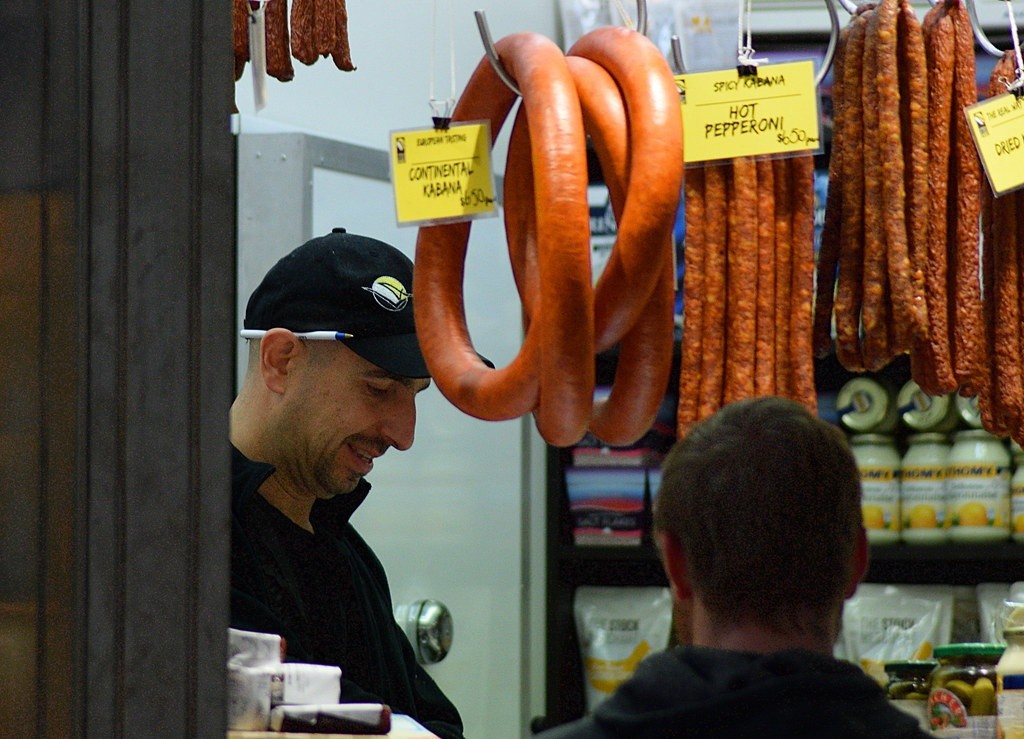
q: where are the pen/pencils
[239,329,355,341]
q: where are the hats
[240,227,496,380]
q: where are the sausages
[233,0,356,82]
[412,1,1024,449]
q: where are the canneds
[880,626,1024,739]
[835,375,1024,546]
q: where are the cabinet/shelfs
[522,149,1024,739]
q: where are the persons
[229,227,495,739]
[531,394,937,739]
